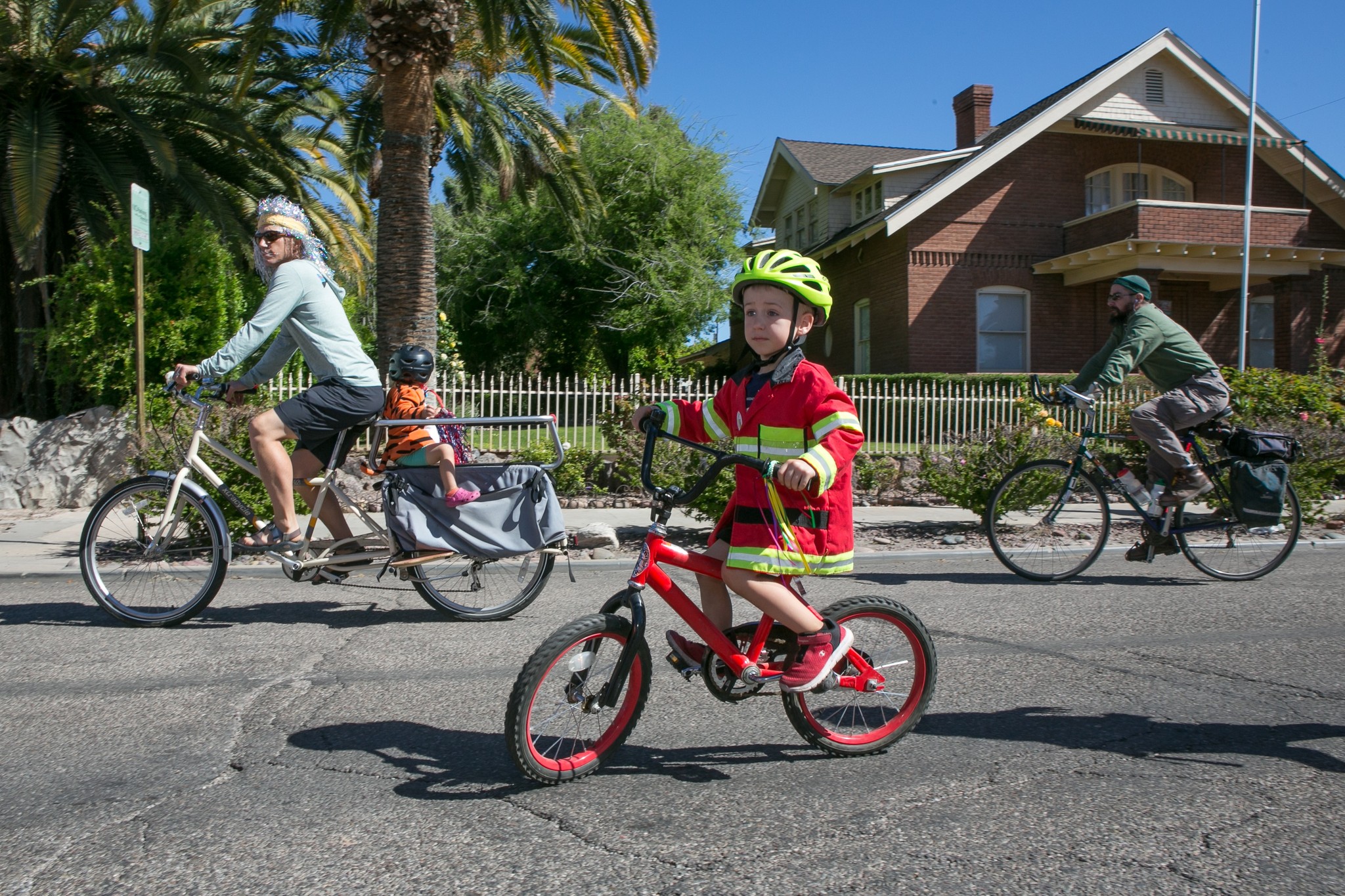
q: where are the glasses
[253,230,287,245]
[1107,293,1136,301]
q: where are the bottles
[1117,467,1152,505]
[1147,478,1167,518]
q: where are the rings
[175,373,180,375]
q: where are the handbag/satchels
[1222,428,1301,464]
[1227,458,1289,525]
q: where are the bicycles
[503,408,939,790]
[79,372,578,629]
[983,374,1303,583]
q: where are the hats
[1112,274,1152,300]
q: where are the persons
[174,196,392,585]
[359,342,482,508]
[633,251,864,693]
[1055,273,1231,561]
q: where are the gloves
[1056,384,1076,400]
[1072,384,1105,411]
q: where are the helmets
[388,344,435,384]
[731,248,834,327]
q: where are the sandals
[311,544,375,586]
[444,486,480,508]
[233,520,305,552]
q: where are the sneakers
[778,617,855,694]
[1125,532,1180,560]
[1157,461,1214,507]
[665,629,737,682]
